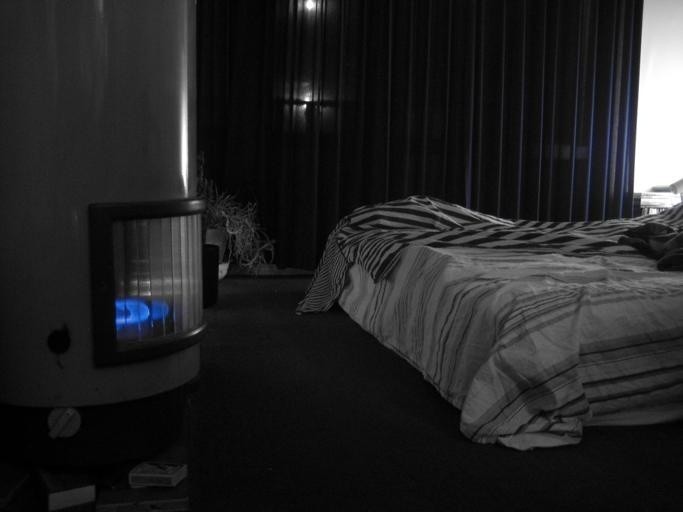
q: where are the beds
[294,193,682,453]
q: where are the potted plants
[201,185,274,277]
